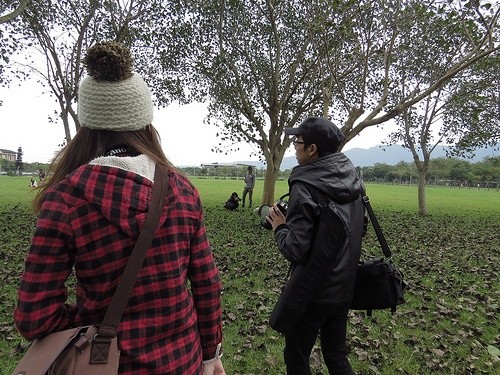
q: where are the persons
[14,42,225,375]
[30,178,37,188]
[224,192,239,210]
[266,117,368,375]
[38,169,45,181]
[242,166,256,208]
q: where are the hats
[77,40,154,131]
[285,116,346,153]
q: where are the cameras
[262,200,289,230]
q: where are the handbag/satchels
[350,258,406,317]
[11,326,121,375]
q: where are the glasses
[293,139,308,146]
